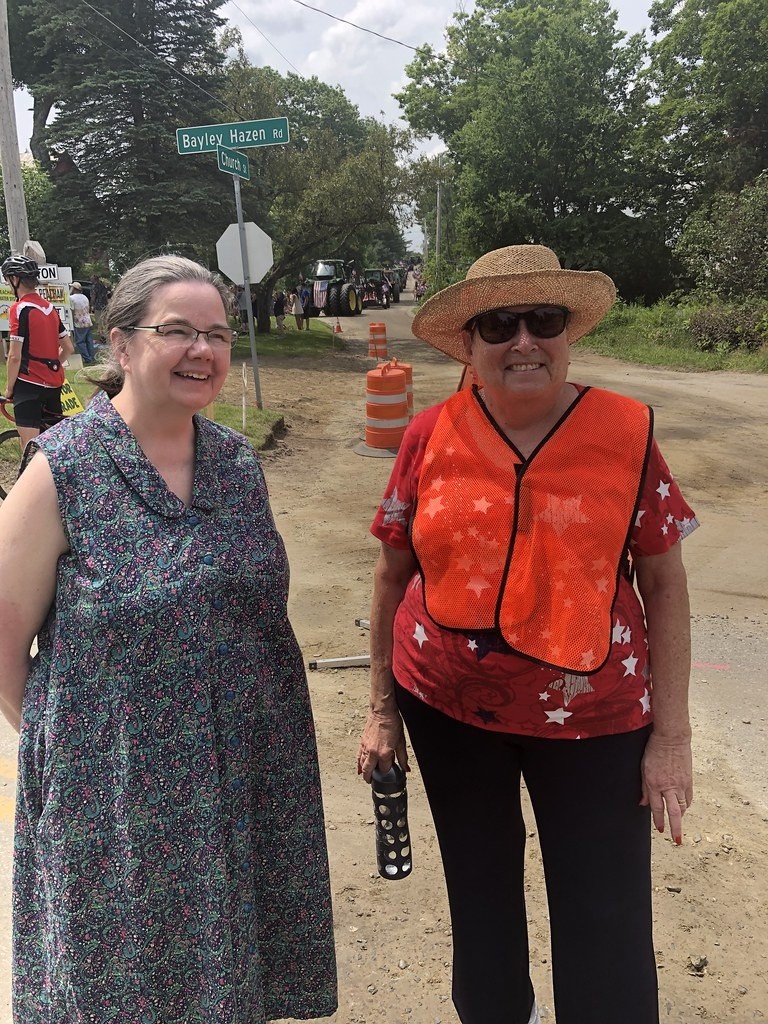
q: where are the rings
[363,750,368,756]
[678,799,687,806]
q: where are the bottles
[371,762,412,880]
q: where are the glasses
[125,323,240,350]
[469,304,573,344]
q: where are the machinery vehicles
[356,259,414,309]
[299,257,365,319]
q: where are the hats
[301,283,305,286]
[69,282,81,290]
[410,244,616,365]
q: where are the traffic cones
[334,316,344,334]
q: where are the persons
[1,331,9,359]
[241,287,305,332]
[301,283,310,330]
[0,254,75,455]
[414,281,428,302]
[69,282,97,366]
[0,255,341,1024]
[354,243,698,1024]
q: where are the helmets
[1,255,40,279]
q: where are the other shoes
[240,332,247,336]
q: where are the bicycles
[0,393,70,503]
